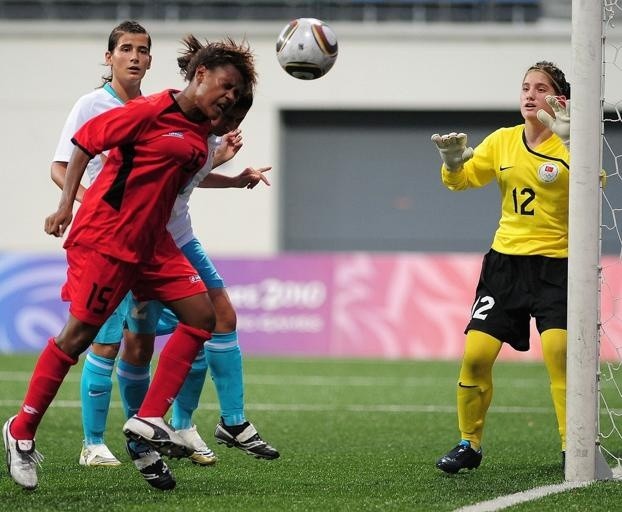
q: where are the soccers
[276,18,338,80]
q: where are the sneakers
[2,414,45,490]
[79,440,123,466]
[437,439,483,474]
[122,414,195,461]
[167,418,218,466]
[214,416,280,460]
[125,438,176,490]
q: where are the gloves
[536,95,570,153]
[430,132,474,171]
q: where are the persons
[117,90,278,489]
[431,62,606,473]
[3,37,256,489]
[51,22,219,467]
